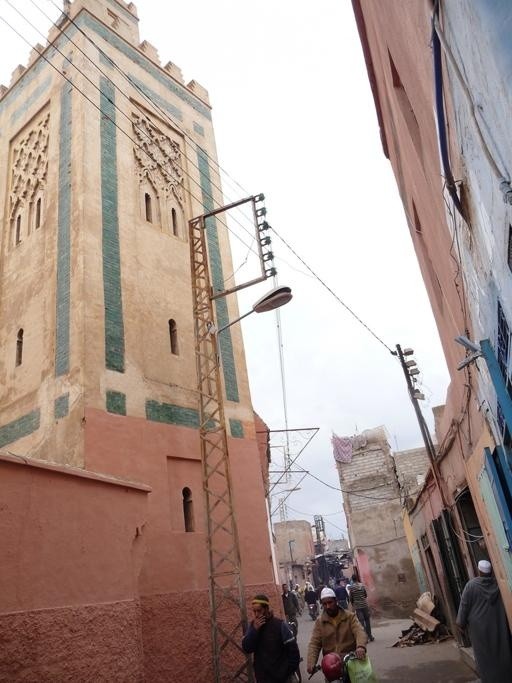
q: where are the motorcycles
[309,598,320,621]
[308,649,357,682]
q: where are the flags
[331,432,352,463]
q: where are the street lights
[394,340,472,596]
[192,282,296,681]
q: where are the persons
[242,595,300,683]
[292,575,352,615]
[350,574,374,641]
[456,559,512,683]
[306,588,366,673]
[281,584,302,629]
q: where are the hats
[478,560,493,574]
[252,594,270,607]
[320,586,337,600]
